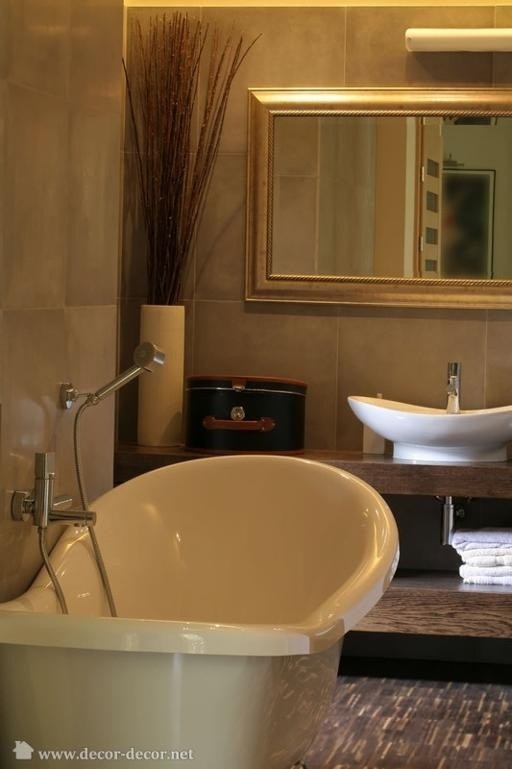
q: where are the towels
[453,528,512,588]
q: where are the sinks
[347,395,512,463]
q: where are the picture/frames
[441,168,496,280]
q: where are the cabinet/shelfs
[120,443,512,649]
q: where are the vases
[139,303,188,449]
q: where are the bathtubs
[1,456,401,768]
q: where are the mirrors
[245,85,512,314]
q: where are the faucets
[446,363,463,412]
[49,507,97,528]
